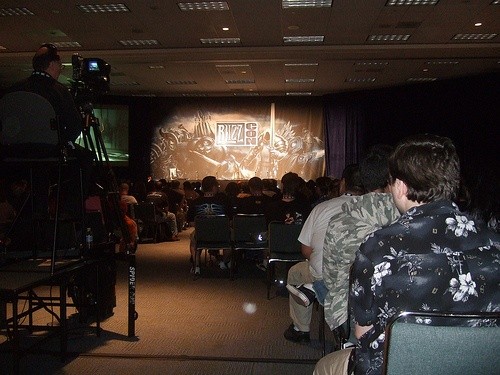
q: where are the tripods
[79,112,135,254]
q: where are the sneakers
[284,321,311,344]
[286,283,316,308]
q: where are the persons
[0,44,470,346]
[313,135,500,375]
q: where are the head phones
[39,44,60,60]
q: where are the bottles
[86,228,93,249]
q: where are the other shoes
[190,265,200,275]
[170,235,180,241]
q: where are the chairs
[231,213,270,279]
[382,310,500,375]
[267,222,305,299]
[193,214,232,276]
[126,200,166,245]
[0,91,85,275]
